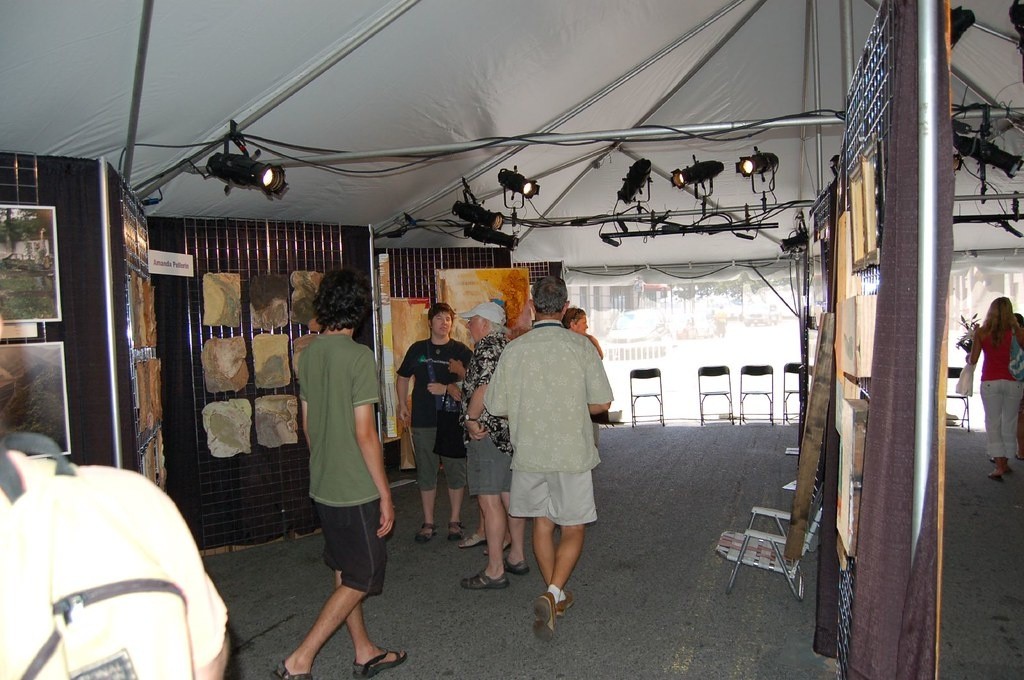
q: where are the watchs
[465,413,477,423]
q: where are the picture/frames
[0,203,63,323]
[0,341,72,461]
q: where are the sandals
[447,521,465,539]
[270,660,313,680]
[415,523,437,543]
[503,554,529,576]
[460,569,510,589]
[352,647,406,680]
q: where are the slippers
[988,473,1004,482]
[483,540,511,556]
[1015,454,1024,460]
[1003,467,1012,475]
[458,534,485,548]
[990,458,996,463]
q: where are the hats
[458,302,506,326]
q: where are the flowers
[955,313,982,352]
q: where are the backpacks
[1,433,195,680]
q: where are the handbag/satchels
[1008,324,1024,380]
[432,385,467,458]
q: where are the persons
[483,275,614,639]
[396,303,475,543]
[269,268,408,680]
[1,430,232,680]
[965,297,1024,480]
[561,307,604,445]
[447,302,530,590]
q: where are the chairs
[697,365,735,426]
[740,365,774,426]
[715,482,824,602]
[947,366,971,432]
[629,367,665,427]
[783,362,799,425]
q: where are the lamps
[451,201,504,230]
[206,135,290,198]
[950,5,976,49]
[954,132,1024,177]
[497,168,540,198]
[1009,4,1024,56]
[617,158,653,213]
[669,154,727,206]
[462,222,519,251]
[734,145,780,204]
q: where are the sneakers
[555,590,574,618]
[533,592,556,641]
